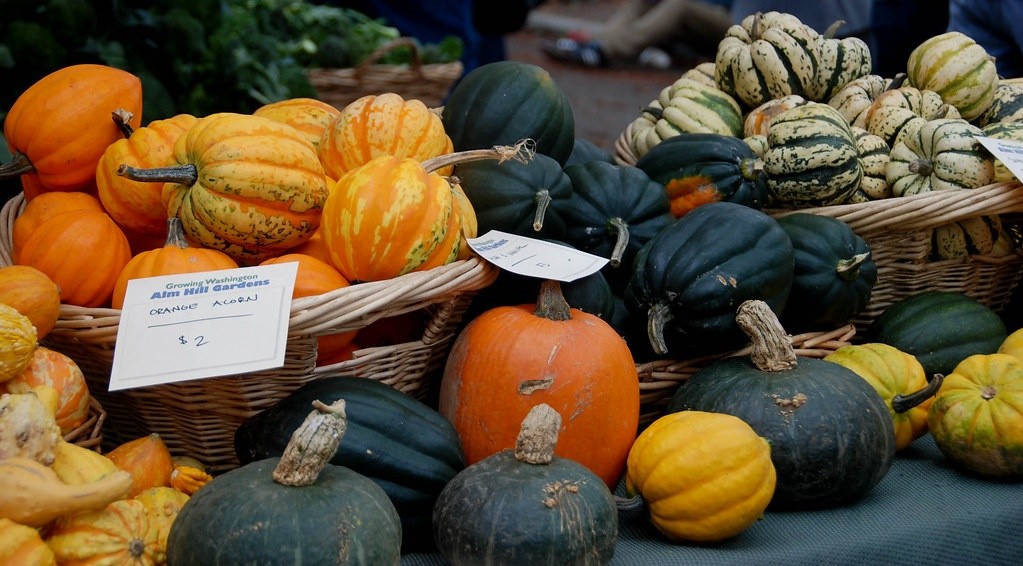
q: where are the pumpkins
[0,10,1023,566]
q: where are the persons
[545,0,733,88]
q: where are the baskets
[623,324,857,432]
[0,191,501,479]
[615,114,1023,323]
[302,39,461,108]
